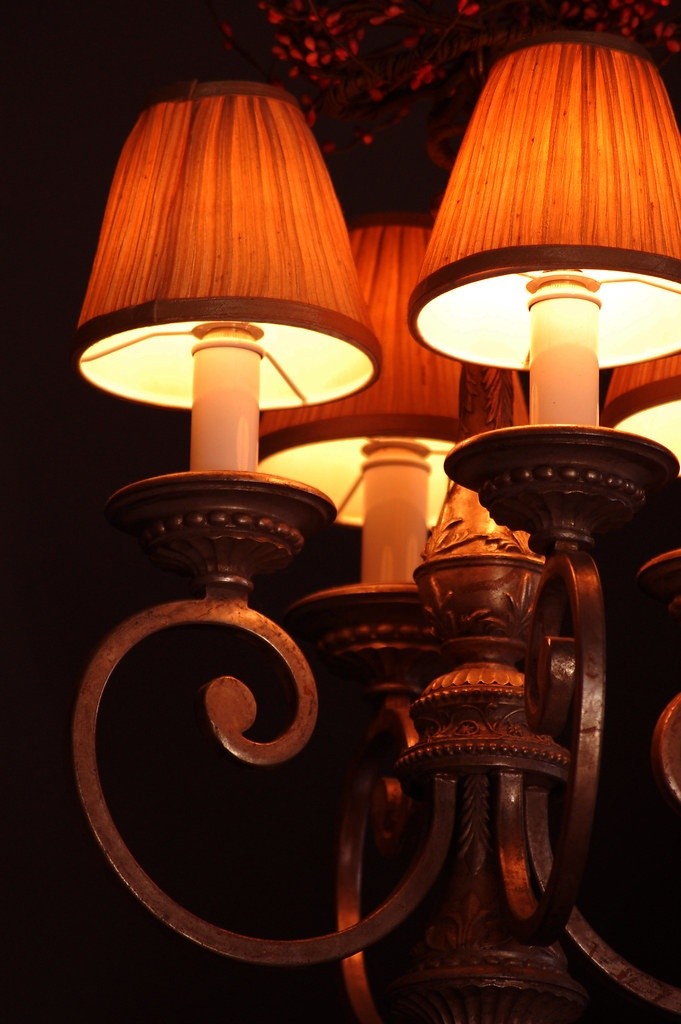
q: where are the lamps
[400,32,681,550]
[254,213,530,700]
[62,77,379,471]
[600,349,681,603]
